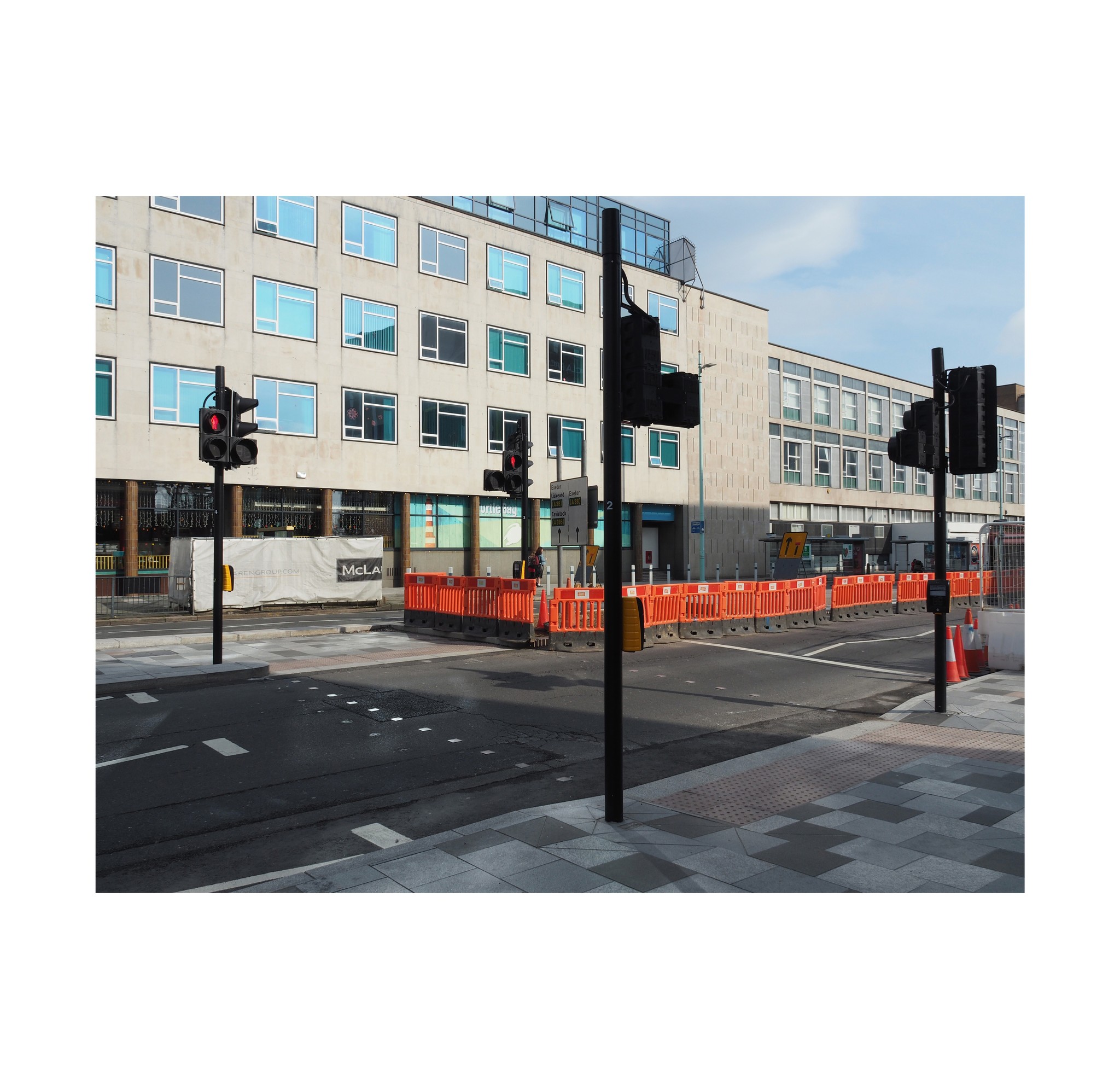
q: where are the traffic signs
[549,476,587,547]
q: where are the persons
[911,559,917,573]
[527,551,534,579]
[534,547,548,587]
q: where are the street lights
[997,426,1015,609]
[698,350,718,584]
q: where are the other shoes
[536,583,538,586]
[537,585,543,587]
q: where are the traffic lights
[199,407,231,463]
[230,390,259,465]
[484,452,524,494]
[522,440,534,488]
[660,369,700,429]
[888,400,928,467]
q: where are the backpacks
[531,555,539,566]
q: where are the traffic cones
[929,608,989,683]
[535,590,550,628]
[566,578,572,588]
